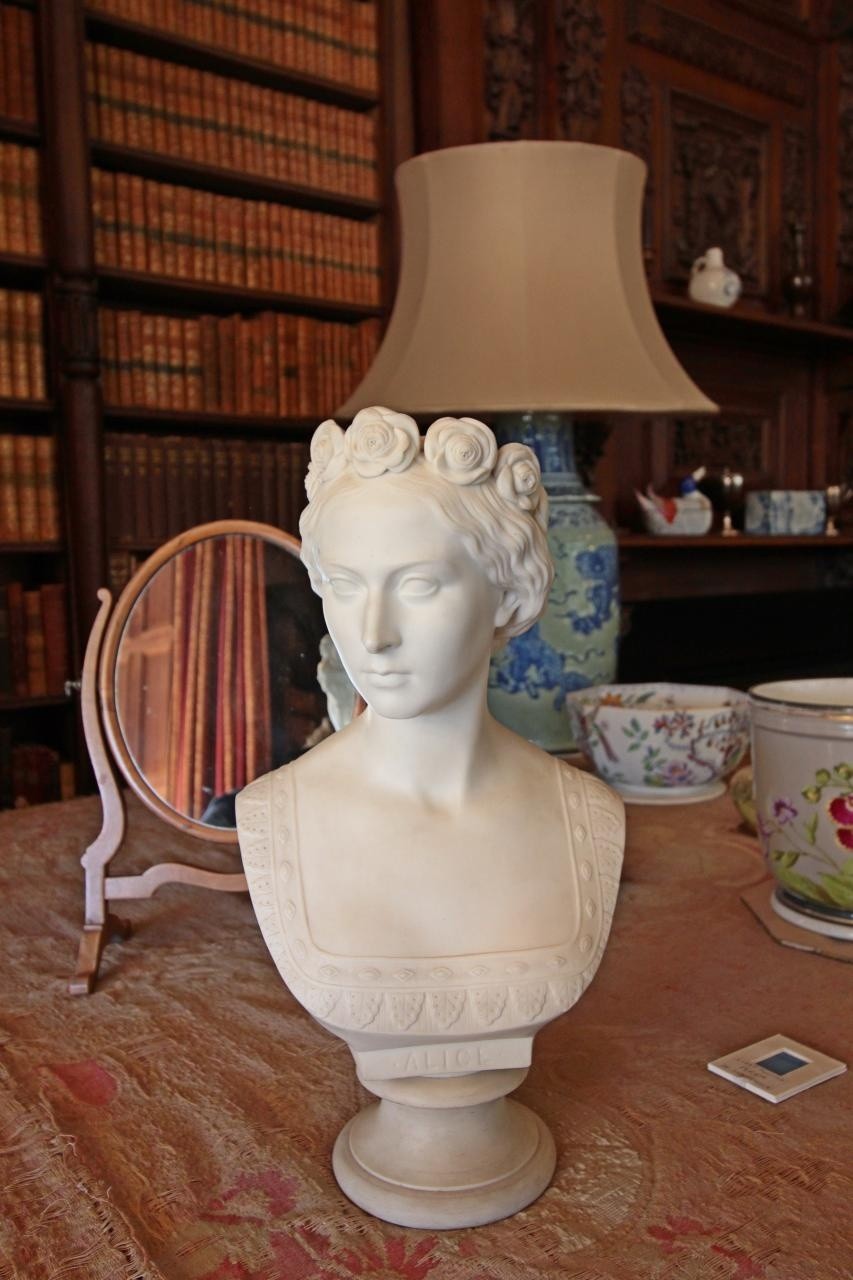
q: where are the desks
[0,753,853,1280]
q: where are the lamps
[330,140,721,761]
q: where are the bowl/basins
[570,684,753,807]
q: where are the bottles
[682,478,702,498]
[776,231,814,309]
[689,247,743,308]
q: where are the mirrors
[68,518,366,996]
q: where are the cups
[750,678,852,938]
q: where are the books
[1,2,75,699]
[85,2,378,593]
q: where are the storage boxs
[744,490,825,536]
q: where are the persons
[234,406,627,1080]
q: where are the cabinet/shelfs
[0,0,401,820]
[614,287,853,602]
